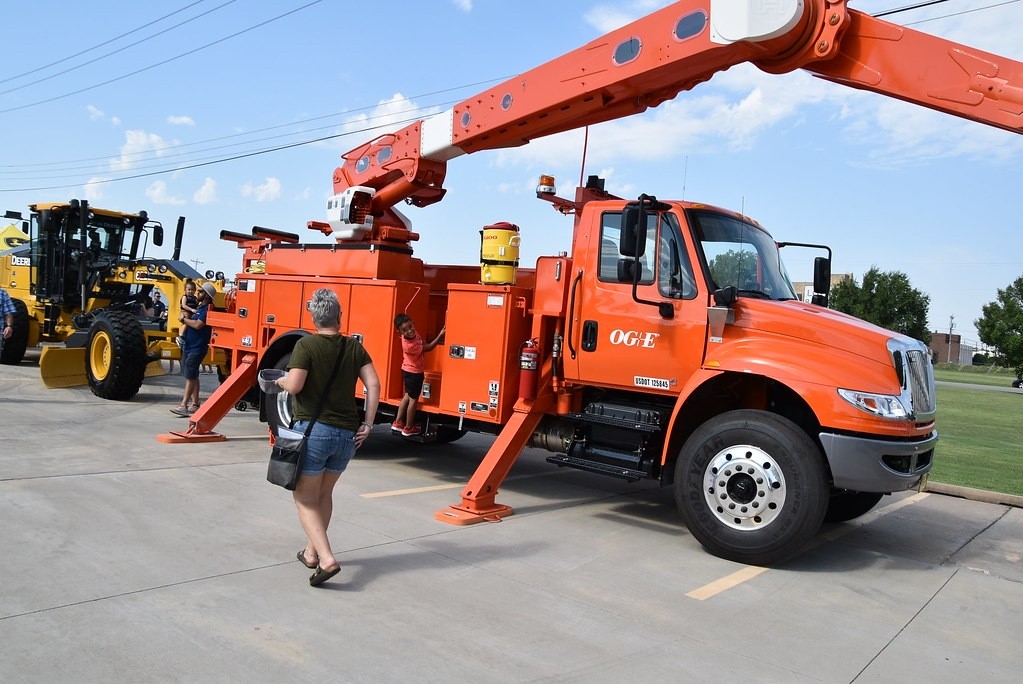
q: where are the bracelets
[362,422,374,429]
[181,317,184,323]
[7,325,12,328]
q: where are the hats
[197,282,217,303]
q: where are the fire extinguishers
[517,337,541,399]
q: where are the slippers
[309,563,341,586]
[297,548,318,568]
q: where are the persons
[0,289,17,357]
[170,281,217,416]
[275,289,380,586]
[390,313,445,436]
[140,292,165,318]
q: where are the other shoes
[169,406,189,417]
[175,336,186,349]
[188,403,201,413]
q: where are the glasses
[155,296,161,298]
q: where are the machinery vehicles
[155,0,1023,567]
[0,199,233,401]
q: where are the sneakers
[402,425,422,436]
[390,421,405,431]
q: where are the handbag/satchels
[266,436,306,491]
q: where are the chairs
[599,239,691,294]
